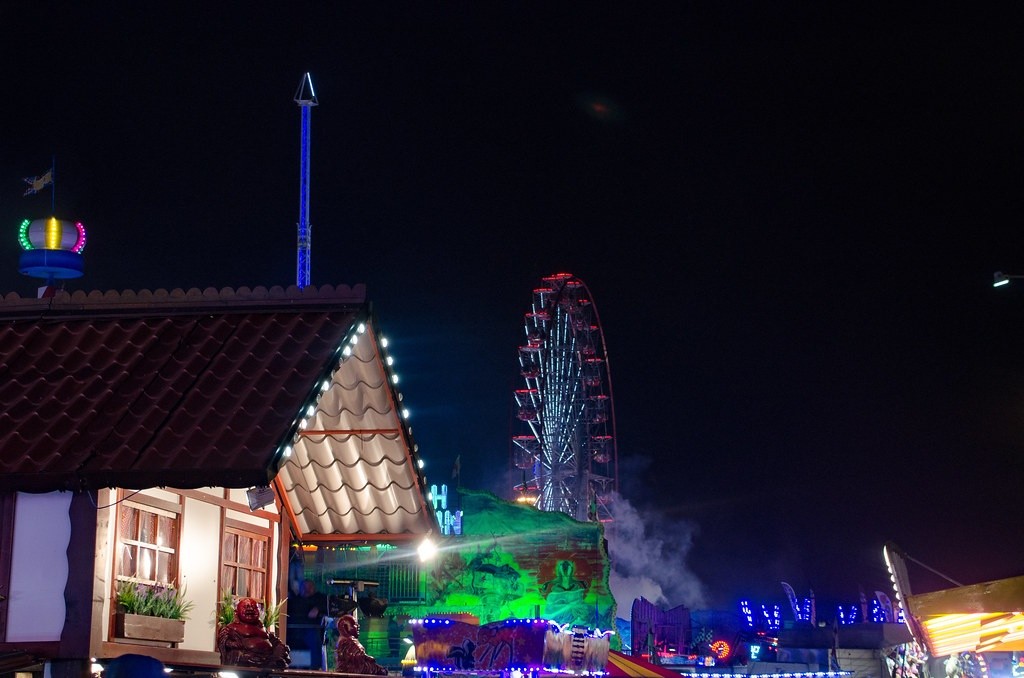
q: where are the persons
[335,614,388,675]
[220,598,292,672]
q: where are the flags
[21,165,54,198]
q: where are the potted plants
[116,572,196,642]
